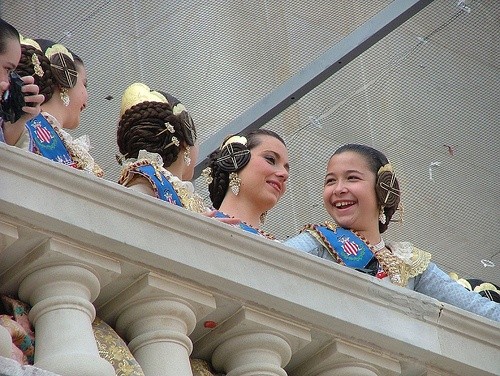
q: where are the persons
[0,19,500,324]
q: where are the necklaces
[372,236,386,253]
